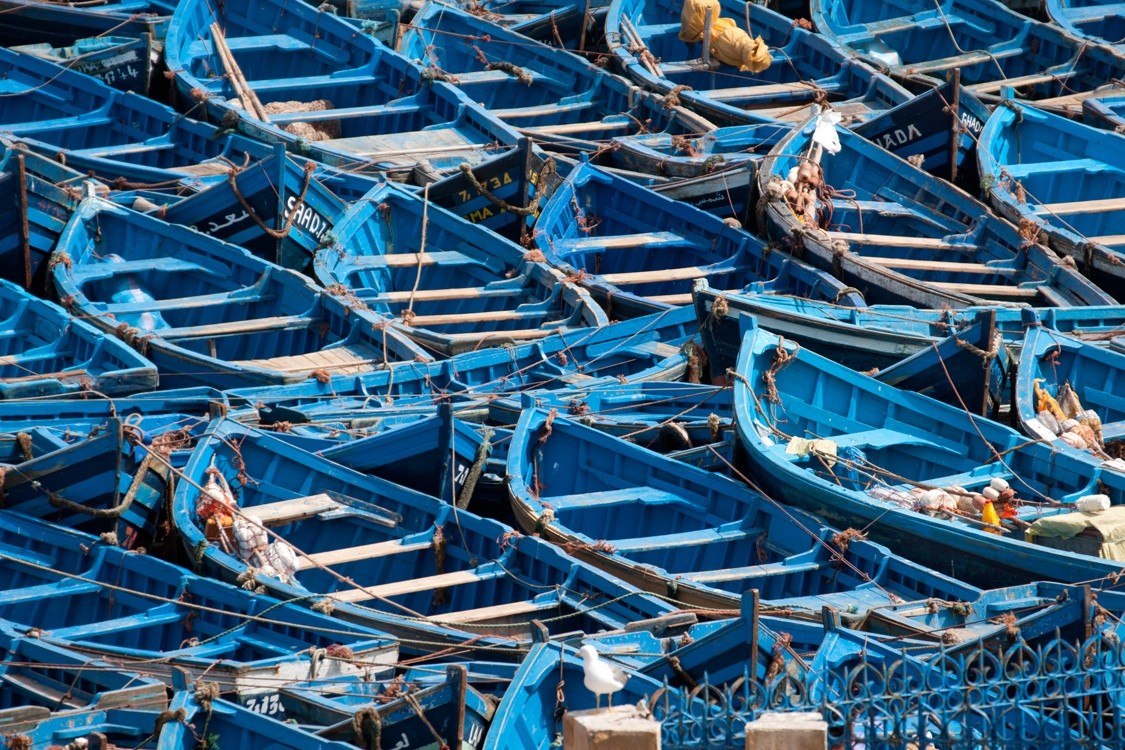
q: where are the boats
[170,398,682,661]
[0,707,168,750]
[0,0,1125,750]
[507,390,988,621]
[157,664,365,749]
[734,306,1124,592]
[483,587,1114,748]
[1,506,401,708]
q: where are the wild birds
[575,644,631,715]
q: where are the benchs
[333,247,576,341]
[629,22,885,122]
[799,157,1125,297]
[0,0,181,160]
[838,2,1125,111]
[768,421,1125,524]
[187,33,639,166]
[0,491,566,661]
[538,484,895,610]
[0,253,386,392]
[549,230,791,303]
